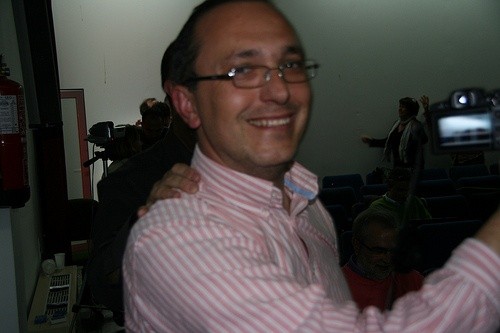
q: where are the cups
[54,253,66,266]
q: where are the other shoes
[98,321,125,333]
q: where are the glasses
[360,239,400,258]
[176,58,320,90]
[395,186,408,193]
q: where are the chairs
[318,165,500,266]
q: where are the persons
[340,95,473,313]
[90,37,201,333]
[122,0,500,333]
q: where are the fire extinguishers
[0,54,32,211]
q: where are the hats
[384,168,418,184]
[399,97,420,116]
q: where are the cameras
[431,88,500,155]
